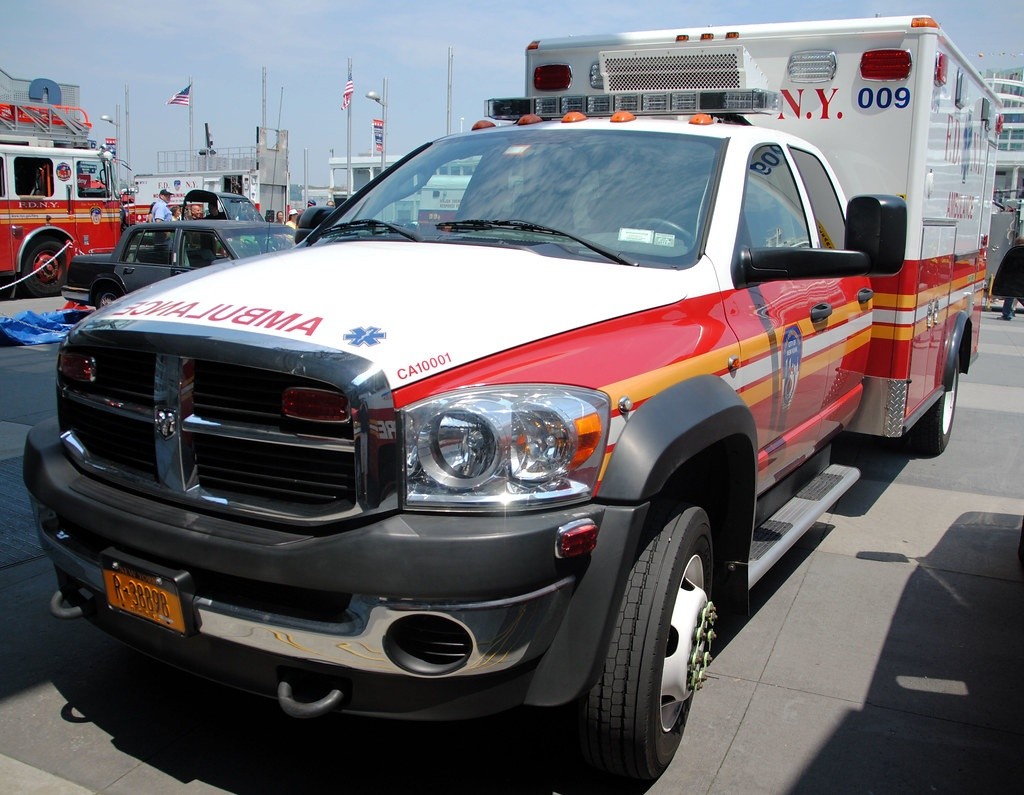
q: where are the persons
[170,204,227,223]
[275,200,334,230]
[151,189,173,224]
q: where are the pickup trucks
[60,218,298,310]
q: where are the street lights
[98,113,120,194]
[364,91,386,172]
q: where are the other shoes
[997,316,1012,321]
[1012,312,1015,317]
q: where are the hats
[160,189,174,195]
[308,200,316,206]
[289,209,298,215]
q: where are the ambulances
[21,7,1006,788]
[417,168,475,229]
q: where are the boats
[971,46,1023,305]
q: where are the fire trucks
[0,98,123,297]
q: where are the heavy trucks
[119,167,260,225]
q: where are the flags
[341,74,354,110]
[167,84,189,105]
